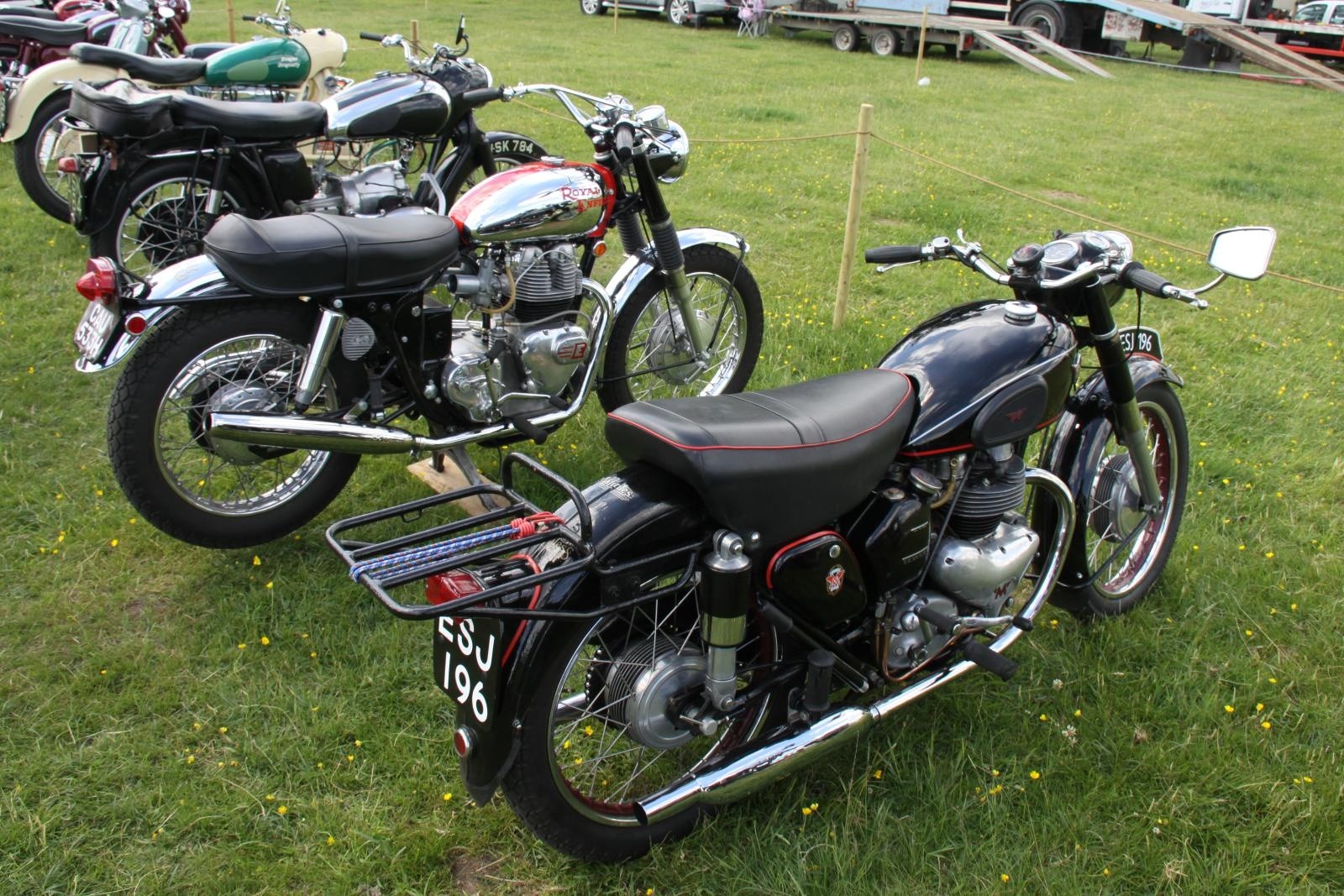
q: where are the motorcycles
[0,1,1279,853]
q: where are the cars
[1281,1,1344,28]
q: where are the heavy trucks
[809,0,1343,98]
